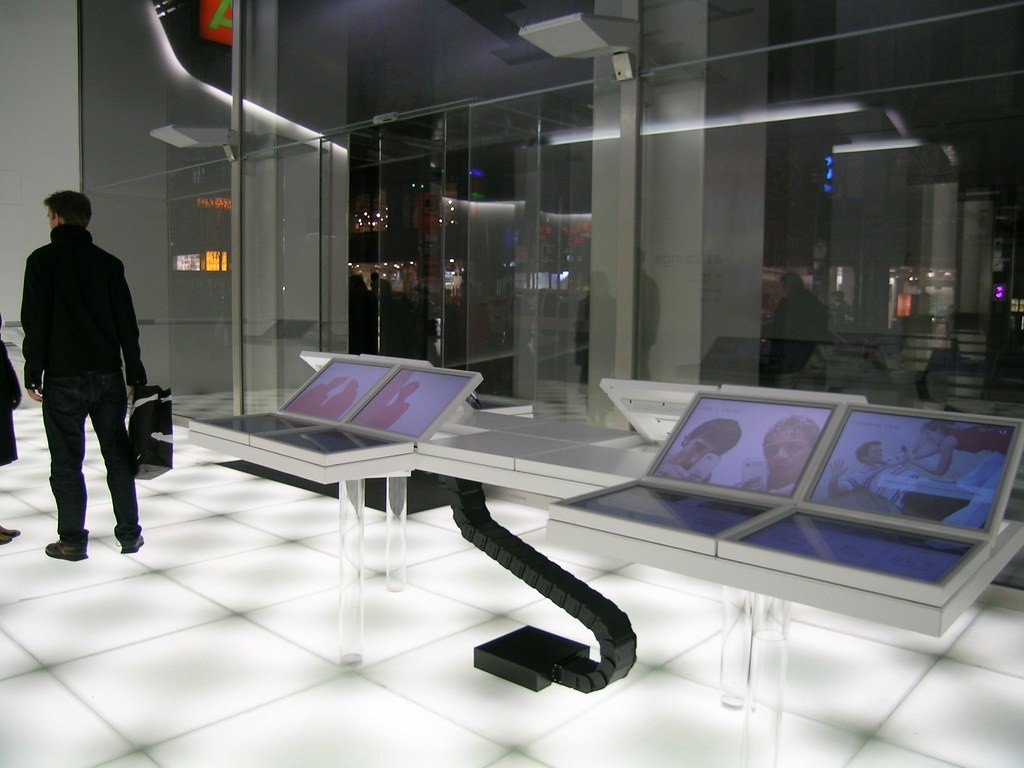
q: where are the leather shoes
[0,524,19,545]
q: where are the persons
[905,420,1016,476]
[827,431,975,519]
[743,414,821,495]
[20,192,151,563]
[767,273,831,374]
[288,376,359,420]
[0,338,20,548]
[363,381,421,430]
[654,417,743,485]
[574,271,617,428]
[632,244,661,382]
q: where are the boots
[114,525,145,554]
[46,530,89,560]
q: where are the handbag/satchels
[129,384,175,480]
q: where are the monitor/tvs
[544,376,1023,609]
[192,351,484,469]
